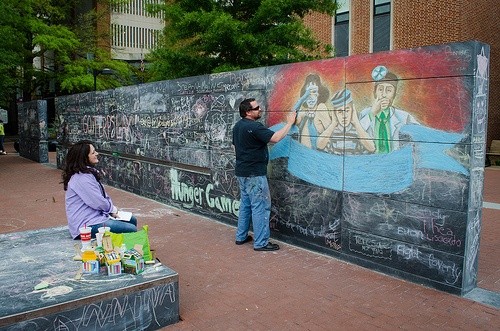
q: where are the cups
[95,232,103,245]
[79,227,91,246]
[98,227,110,231]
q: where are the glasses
[250,105,259,110]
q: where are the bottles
[120,244,126,255]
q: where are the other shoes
[236,235,253,245]
[254,241,280,250]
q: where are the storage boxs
[82,244,145,276]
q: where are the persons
[0,119,7,155]
[232,98,298,251]
[58,141,138,240]
